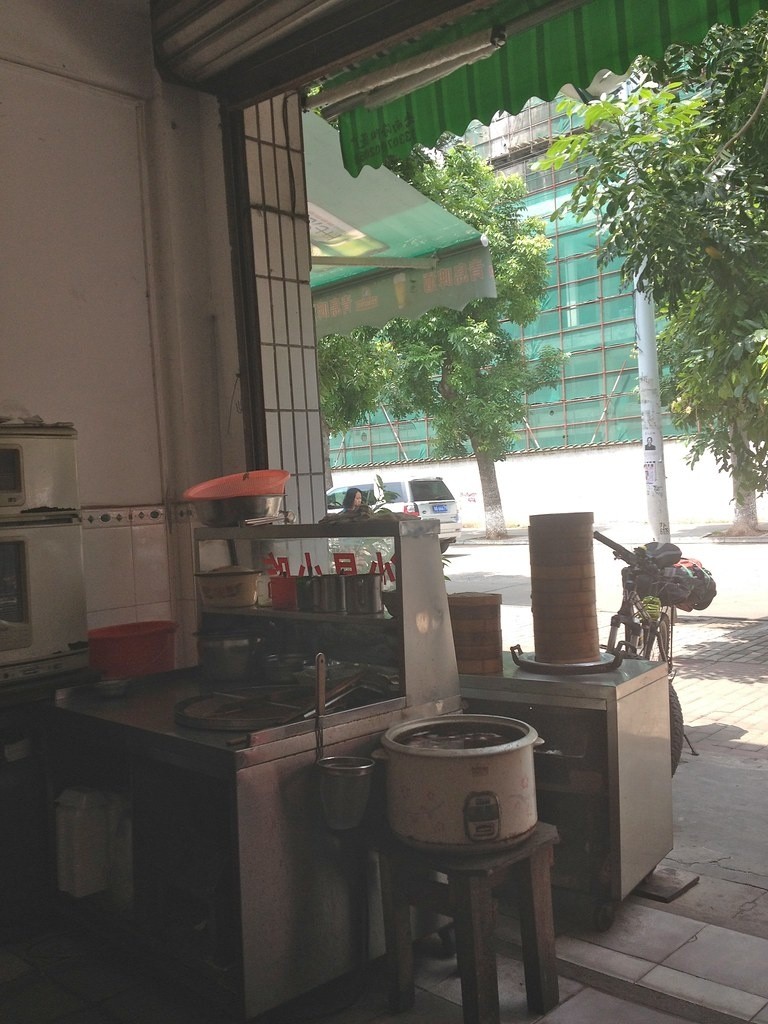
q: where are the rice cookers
[376,713,545,853]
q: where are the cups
[306,573,344,613]
[268,576,296,610]
[296,576,317,612]
[345,573,383,614]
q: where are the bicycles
[593,530,697,780]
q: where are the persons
[338,488,362,514]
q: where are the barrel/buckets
[88,618,176,682]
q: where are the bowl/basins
[184,491,288,529]
[194,563,261,608]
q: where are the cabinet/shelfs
[36,726,233,976]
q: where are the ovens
[1,418,89,683]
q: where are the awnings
[329,0,768,175]
[302,108,496,338]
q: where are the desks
[322,801,560,1024]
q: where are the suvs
[326,478,461,553]
